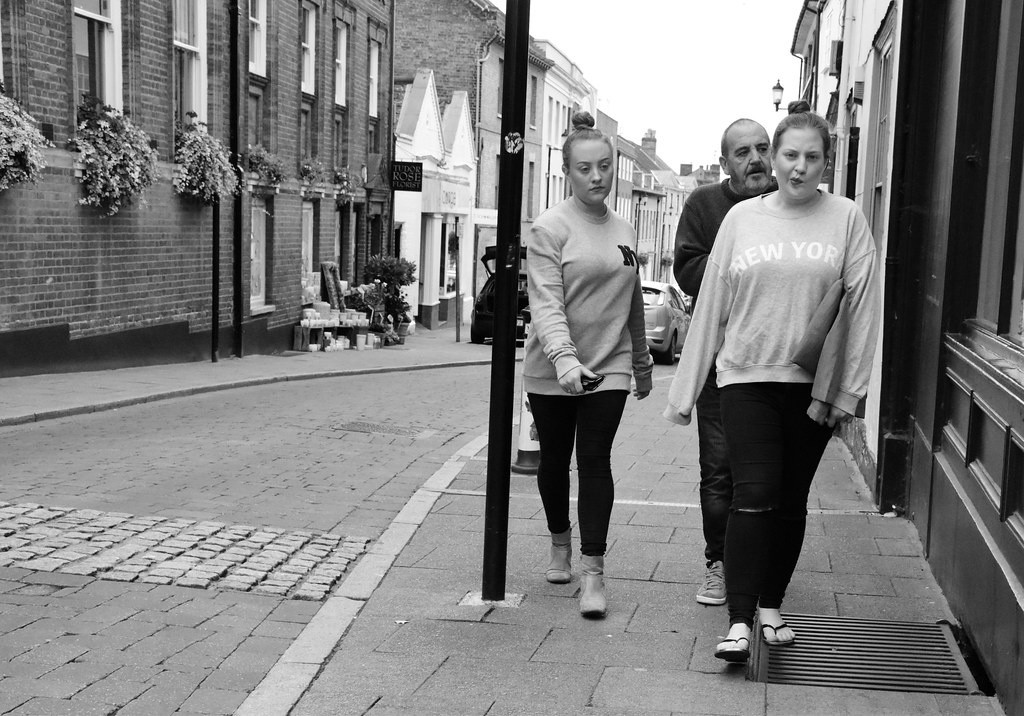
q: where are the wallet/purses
[581,373,605,391]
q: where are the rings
[567,389,571,392]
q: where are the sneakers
[696,560,727,605]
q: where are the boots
[580,554,607,615]
[546,521,573,582]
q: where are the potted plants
[361,252,415,345]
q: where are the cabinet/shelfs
[292,325,369,352]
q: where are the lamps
[772,79,788,112]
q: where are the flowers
[0,78,362,220]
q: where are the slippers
[761,623,794,645]
[714,637,750,662]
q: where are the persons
[674,99,879,662]
[523,112,653,617]
[672,119,779,603]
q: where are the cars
[640,281,691,365]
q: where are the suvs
[470,245,533,344]
